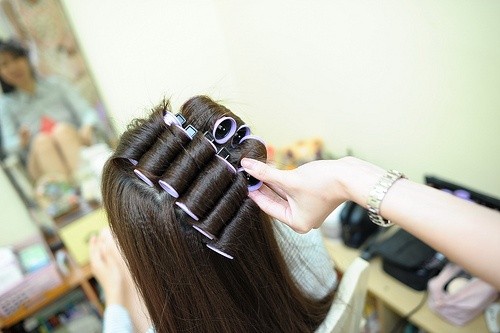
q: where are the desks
[0,264,106,333]
[326,244,491,333]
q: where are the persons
[0,34,103,196]
[236,156,500,291]
[89,96,340,333]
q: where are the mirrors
[0,1,122,241]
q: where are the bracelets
[367,169,407,227]
